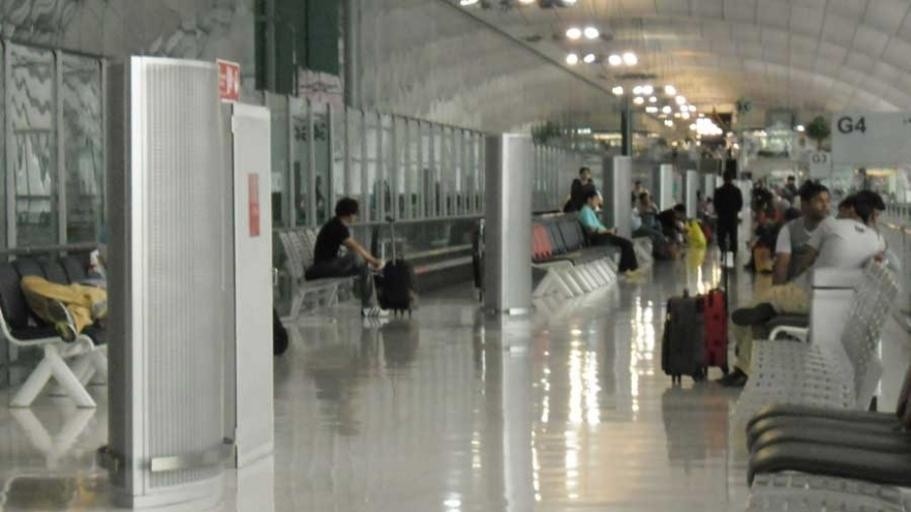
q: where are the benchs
[742,250,909,512]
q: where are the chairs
[525,211,626,299]
[280,226,365,316]
[0,255,108,409]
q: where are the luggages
[660,287,726,374]
[382,260,414,308]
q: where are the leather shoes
[717,368,749,388]
[731,303,775,326]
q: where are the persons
[20,246,108,343]
[315,195,391,318]
